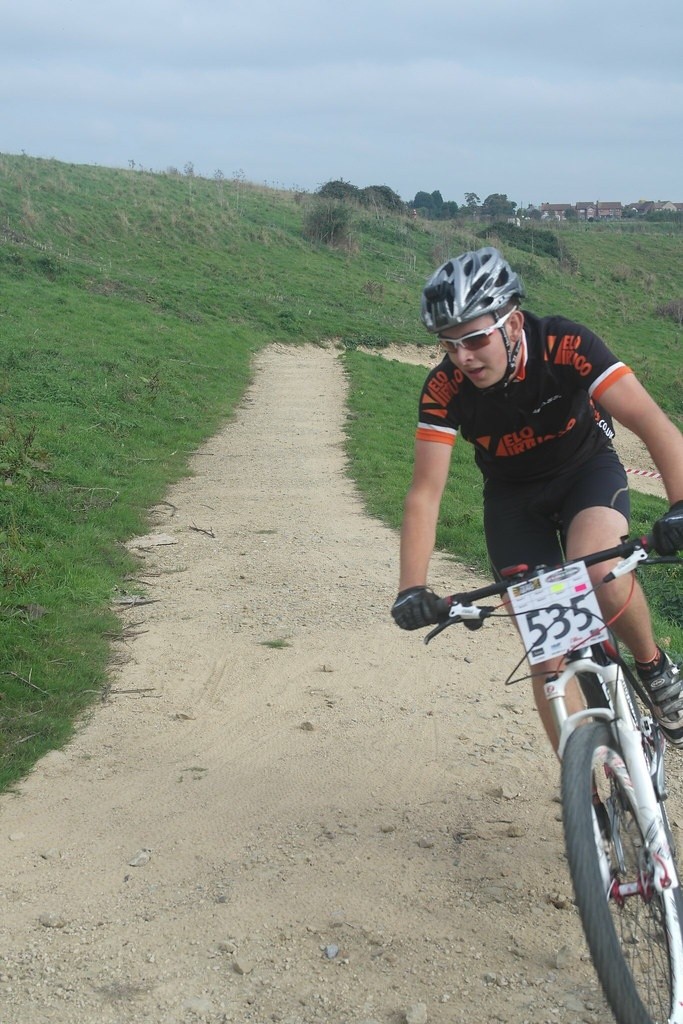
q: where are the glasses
[437,304,517,353]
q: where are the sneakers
[635,644,683,750]
[594,802,611,865]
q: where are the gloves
[391,585,442,630]
[652,499,683,557]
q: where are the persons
[389,248,683,858]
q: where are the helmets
[419,247,525,334]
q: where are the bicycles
[393,507,683,1024]
